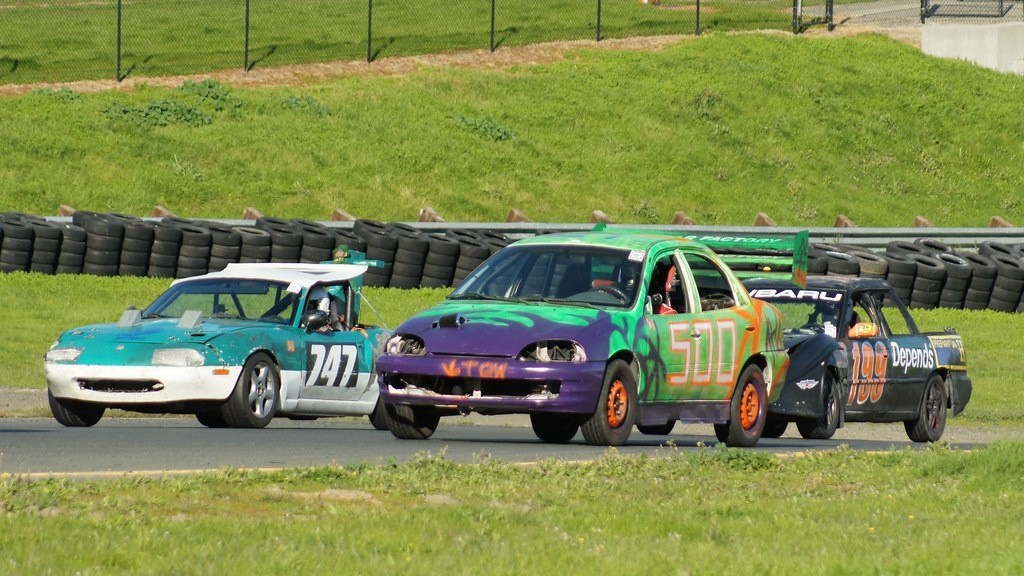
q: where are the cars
[43,243,396,429]
[741,274,972,443]
[374,216,810,447]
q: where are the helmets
[821,298,853,326]
[291,285,330,316]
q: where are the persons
[783,297,853,338]
[594,260,678,314]
[300,287,333,332]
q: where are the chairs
[328,295,343,331]
[561,265,677,317]
[849,310,860,328]
